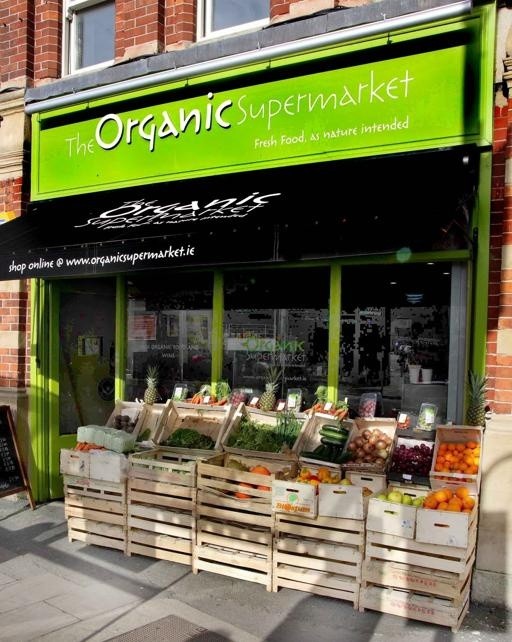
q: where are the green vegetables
[227,411,304,453]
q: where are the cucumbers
[300,424,352,463]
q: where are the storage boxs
[58,447,90,479]
[197,454,282,592]
[126,449,215,577]
[151,398,229,452]
[62,474,126,557]
[358,530,478,634]
[90,449,127,484]
[105,399,143,435]
[272,467,342,519]
[414,505,480,549]
[317,470,387,521]
[342,417,398,476]
[273,512,366,611]
[384,427,437,489]
[134,402,166,448]
[428,423,483,495]
[299,410,355,468]
[221,402,314,458]
[365,479,431,540]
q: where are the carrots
[191,393,203,405]
[214,398,226,405]
[303,403,348,422]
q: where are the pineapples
[465,369,488,426]
[144,366,158,405]
[259,362,283,410]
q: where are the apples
[434,441,480,482]
[423,487,474,513]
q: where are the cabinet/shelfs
[170,400,397,429]
[399,374,448,416]
